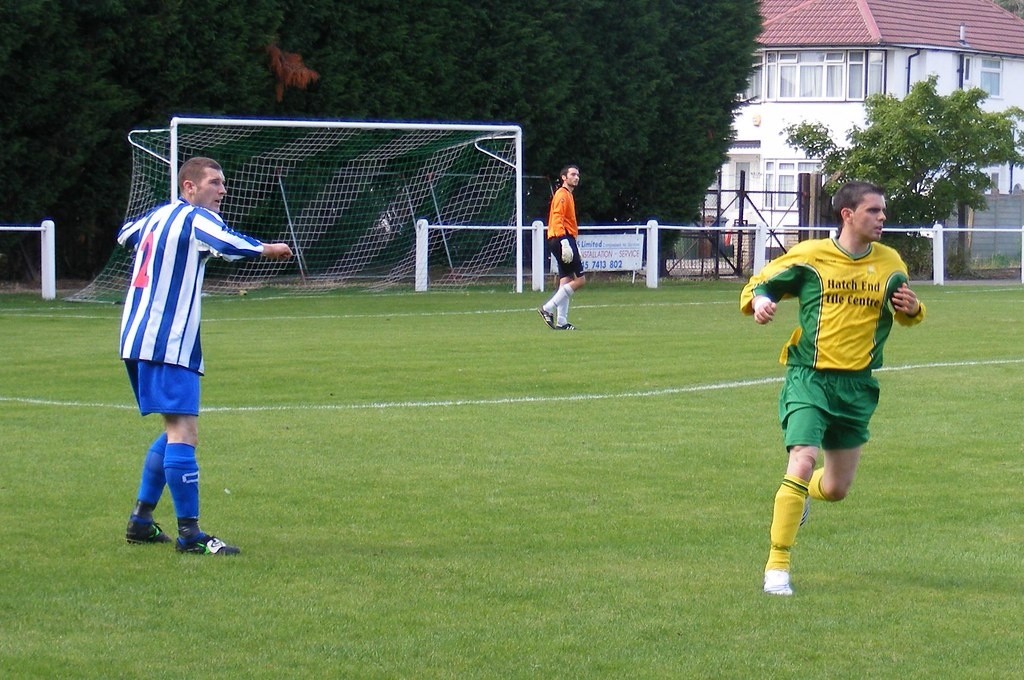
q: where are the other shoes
[799,493,810,526]
[763,569,793,596]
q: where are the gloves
[560,238,574,263]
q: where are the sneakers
[175,532,240,555]
[126,520,173,544]
[555,323,577,330]
[538,306,554,329]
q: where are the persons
[539,165,585,330]
[116,157,293,557]
[741,181,926,596]
